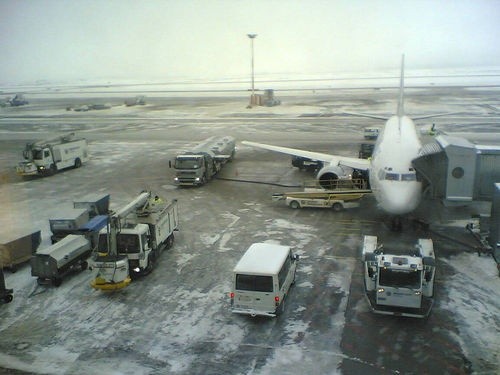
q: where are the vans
[232,240,299,318]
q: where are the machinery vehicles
[272,178,372,212]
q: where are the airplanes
[242,53,500,232]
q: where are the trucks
[87,190,179,291]
[16,133,89,176]
[364,127,381,139]
[32,193,121,286]
[361,231,437,320]
[168,135,235,187]
